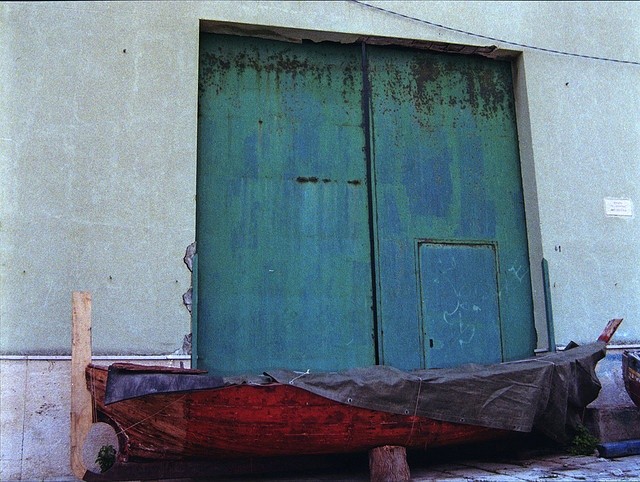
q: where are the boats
[85,340,607,454]
[622,349,640,408]
